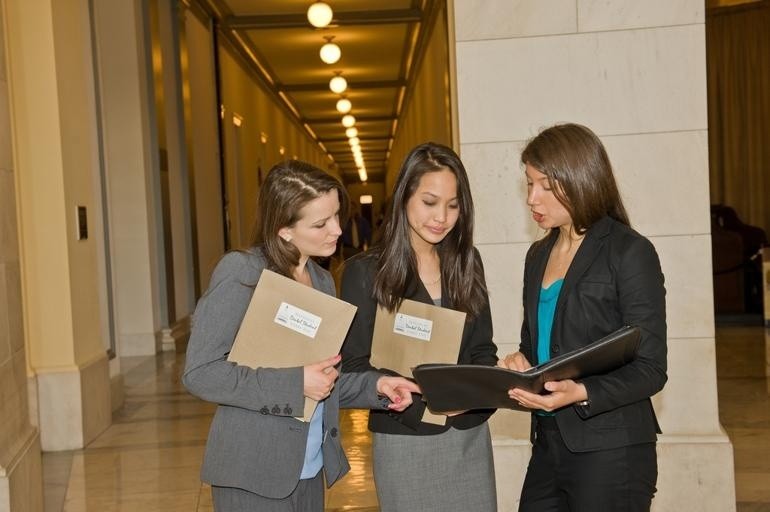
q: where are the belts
[343,243,363,250]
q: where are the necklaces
[421,272,441,285]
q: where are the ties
[351,217,359,249]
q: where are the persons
[494,124,669,512]
[183,160,421,512]
[341,142,499,512]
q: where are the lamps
[329,70,348,93]
[342,115,356,126]
[320,34,340,64]
[306,0,333,28]
[346,128,358,137]
[336,95,352,113]
[348,139,368,181]
